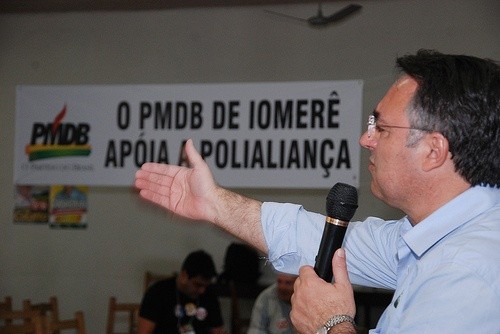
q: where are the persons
[247,273,299,334]
[137,250,228,334]
[13,186,47,209]
[52,185,86,209]
[135,49,500,334]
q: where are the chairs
[1,271,158,334]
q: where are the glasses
[367,114,431,139]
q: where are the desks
[231,285,395,334]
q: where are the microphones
[313,182,359,284]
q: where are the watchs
[316,315,358,334]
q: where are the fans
[262,0,363,27]
